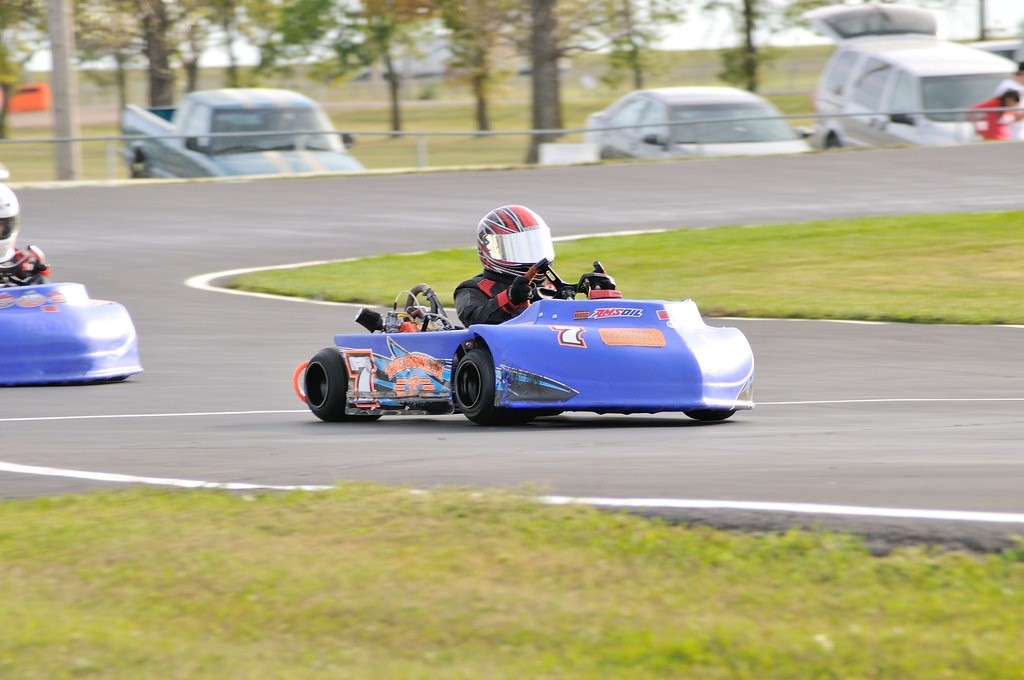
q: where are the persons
[996,61,1024,140]
[453,204,568,329]
[969,89,1023,140]
[0,180,44,288]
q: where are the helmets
[476,205,555,283]
[0,182,19,263]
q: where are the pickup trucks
[119,86,369,181]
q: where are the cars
[796,2,1023,147]
[582,84,824,163]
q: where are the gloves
[588,269,616,290]
[507,276,536,306]
